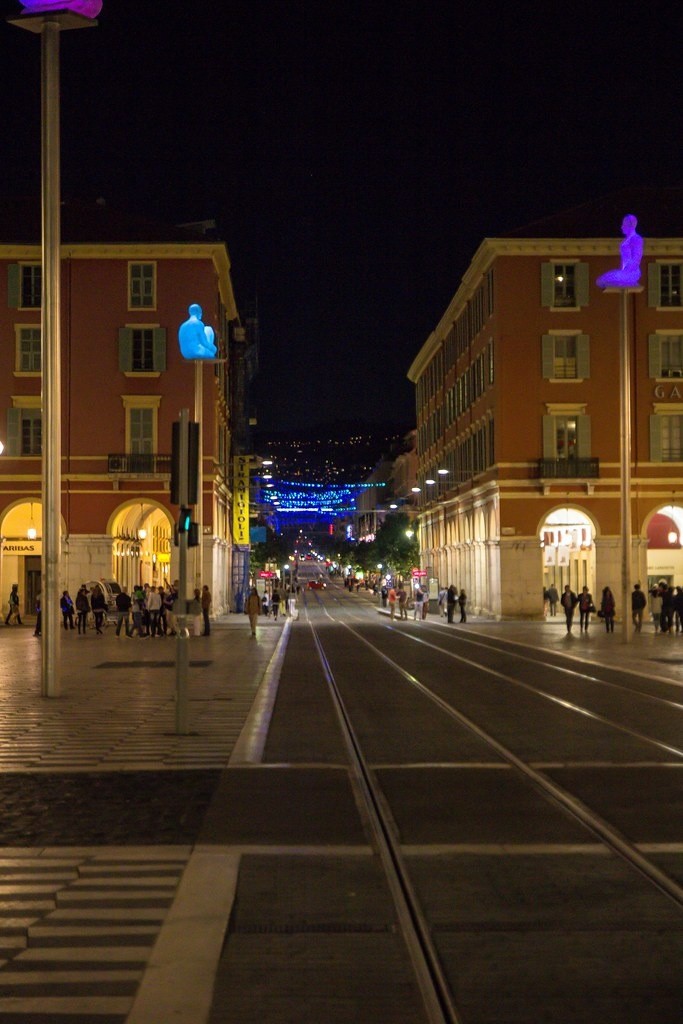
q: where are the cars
[300,552,312,561]
[306,580,326,590]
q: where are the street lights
[594,215,646,645]
[171,303,219,736]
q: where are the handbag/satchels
[439,601,442,606]
[588,603,596,612]
[597,610,605,618]
[261,597,266,602]
[12,605,18,616]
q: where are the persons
[595,215,643,288]
[413,584,429,621]
[544,583,559,616]
[178,303,216,359]
[381,584,388,607]
[243,587,263,638]
[60,590,76,630]
[114,578,213,637]
[648,582,683,633]
[439,584,448,617]
[458,589,467,623]
[5,584,23,626]
[33,588,42,637]
[347,573,379,596]
[260,577,299,621]
[561,585,578,633]
[577,586,593,634]
[396,585,407,621]
[76,583,108,637]
[447,585,457,623]
[602,586,616,633]
[388,586,399,621]
[630,584,647,633]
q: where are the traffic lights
[179,508,192,532]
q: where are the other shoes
[155,633,163,638]
[251,632,256,638]
[168,631,176,637]
[140,634,149,639]
[70,627,75,630]
[275,618,276,621]
[125,635,135,640]
[18,622,23,625]
[115,635,118,639]
[97,627,103,635]
[634,624,683,636]
[202,633,209,637]
[5,621,10,624]
